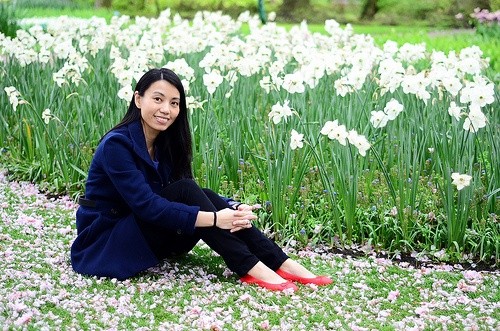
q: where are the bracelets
[214,211,217,226]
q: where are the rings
[247,220,249,224]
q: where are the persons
[71,68,330,290]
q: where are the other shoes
[240,273,298,292]
[275,269,333,286]
